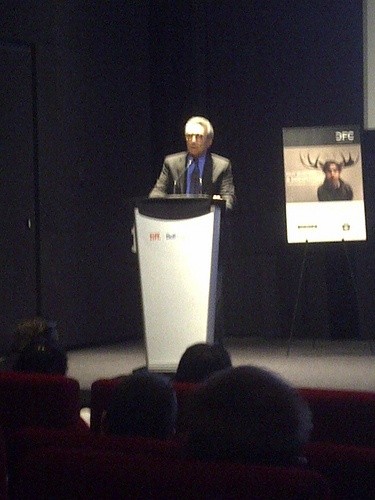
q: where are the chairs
[0,371,375,500]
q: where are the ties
[188,157,203,194]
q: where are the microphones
[173,160,193,194]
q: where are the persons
[173,344,232,385]
[173,366,314,467]
[317,160,354,201]
[100,373,178,442]
[145,116,235,348]
[1,318,91,435]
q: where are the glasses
[185,133,204,141]
